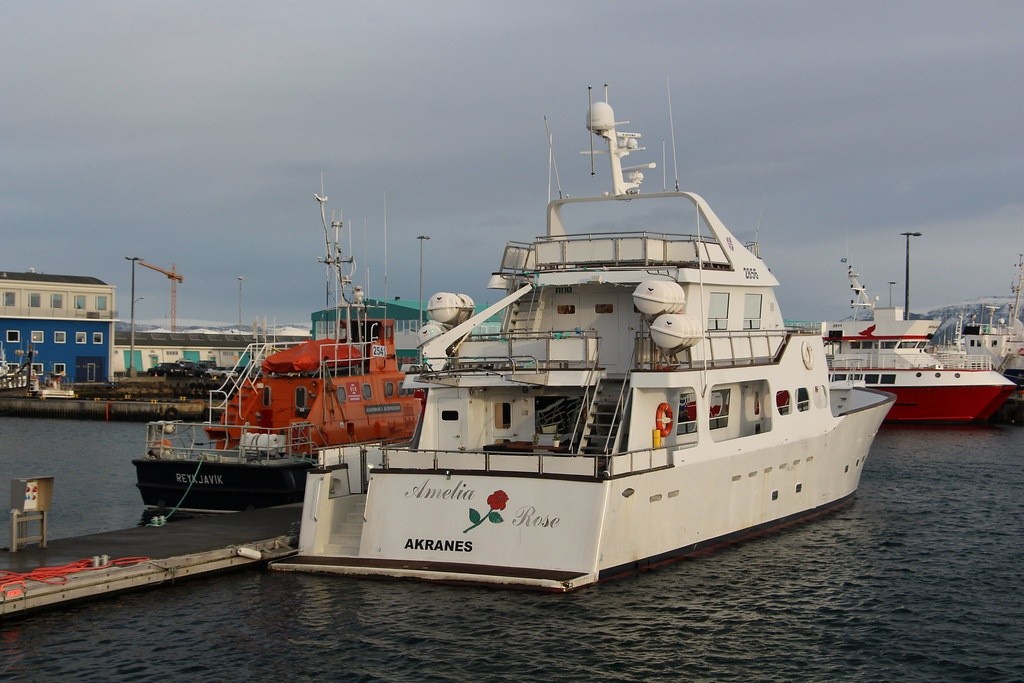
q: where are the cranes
[138,260,184,334]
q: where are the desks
[507,442,568,450]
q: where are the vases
[553,441,560,447]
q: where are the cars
[146,359,263,380]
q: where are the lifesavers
[188,382,220,392]
[801,339,814,370]
[165,407,179,421]
[203,408,216,420]
[656,402,674,437]
[292,428,308,451]
[1019,348,1024,356]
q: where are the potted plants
[532,433,540,445]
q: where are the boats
[265,89,899,595]
[129,171,429,515]
[814,240,1024,425]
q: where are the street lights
[123,256,141,378]
[415,235,434,366]
[888,281,896,307]
[899,230,923,320]
[237,276,243,333]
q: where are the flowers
[552,433,563,441]
